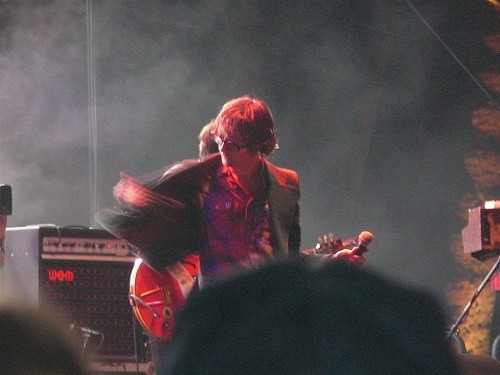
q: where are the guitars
[127,230,361,342]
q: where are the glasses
[214,134,261,153]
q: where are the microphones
[70,323,100,336]
[0,185,11,265]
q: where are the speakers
[6,224,146,364]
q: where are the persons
[116,95,367,292]
[160,121,219,184]
[164,254,465,375]
